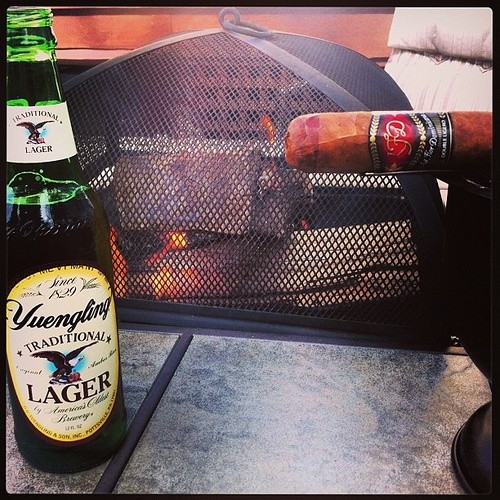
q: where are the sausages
[284,111,494,175]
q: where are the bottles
[1,5,128,474]
